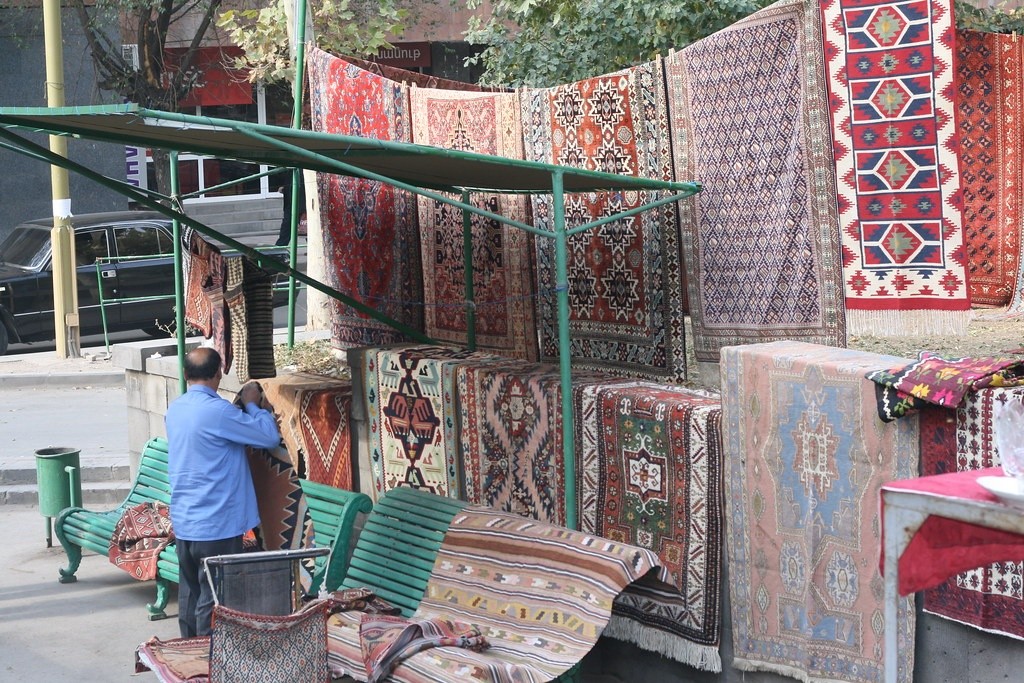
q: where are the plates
[975,476,1024,507]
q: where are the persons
[164,347,280,638]
[276,170,305,246]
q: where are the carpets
[113,0,1023,683]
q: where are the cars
[0,211,300,357]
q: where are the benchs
[337,485,469,616]
[55,436,373,620]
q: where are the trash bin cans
[33,446,83,517]
